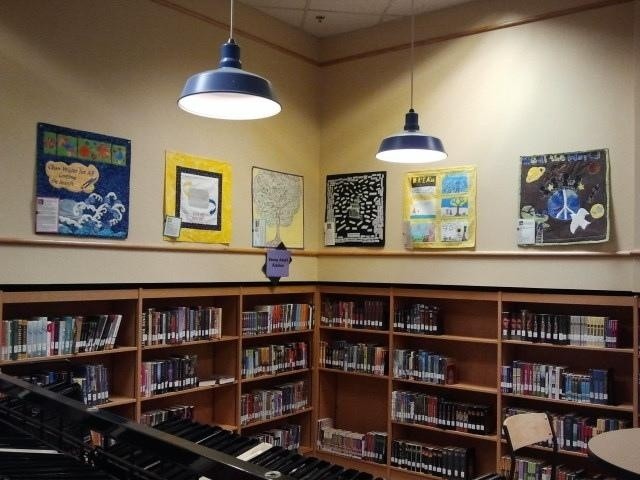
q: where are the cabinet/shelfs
[140,280,241,434]
[390,281,500,478]
[241,279,318,458]
[499,285,640,480]
[1,282,140,426]
[315,280,391,478]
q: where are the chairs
[504,411,556,480]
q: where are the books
[242,304,314,336]
[391,437,470,479]
[241,381,308,451]
[141,351,198,427]
[391,389,496,436]
[319,340,388,376]
[141,305,222,347]
[500,358,617,406]
[501,406,631,480]
[242,341,307,379]
[319,300,387,330]
[2,313,122,406]
[393,300,445,336]
[317,418,388,464]
[502,308,620,349]
[392,347,458,385]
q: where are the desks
[588,425,640,480]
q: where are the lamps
[374,0,451,165]
[177,0,282,122]
